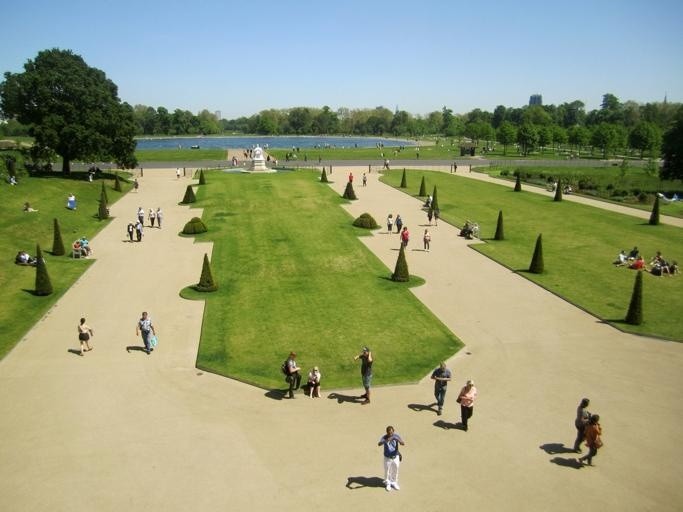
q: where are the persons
[430,363,452,416]
[348,134,425,187]
[552,182,572,194]
[430,134,603,172]
[656,192,679,202]
[354,347,373,405]
[377,425,405,492]
[573,397,590,454]
[612,246,680,278]
[578,414,603,466]
[77,316,94,357]
[455,380,477,431]
[281,351,302,398]
[423,194,440,226]
[229,142,337,168]
[457,220,479,240]
[307,366,321,398]
[6,143,186,267]
[137,311,156,354]
[386,214,431,252]
